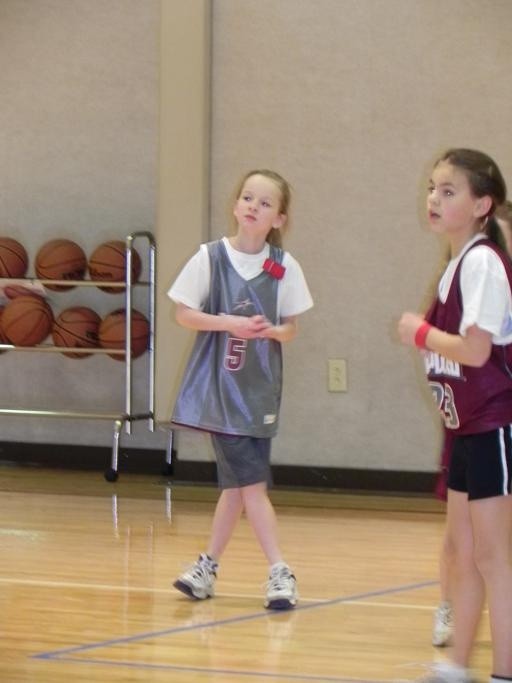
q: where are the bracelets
[415,322,433,351]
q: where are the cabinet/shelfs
[0,223,175,483]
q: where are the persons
[396,148,512,683]
[433,200,512,647]
[165,168,313,611]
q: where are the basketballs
[1,238,151,361]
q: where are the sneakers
[433,604,455,646]
[173,552,220,598]
[263,563,298,610]
